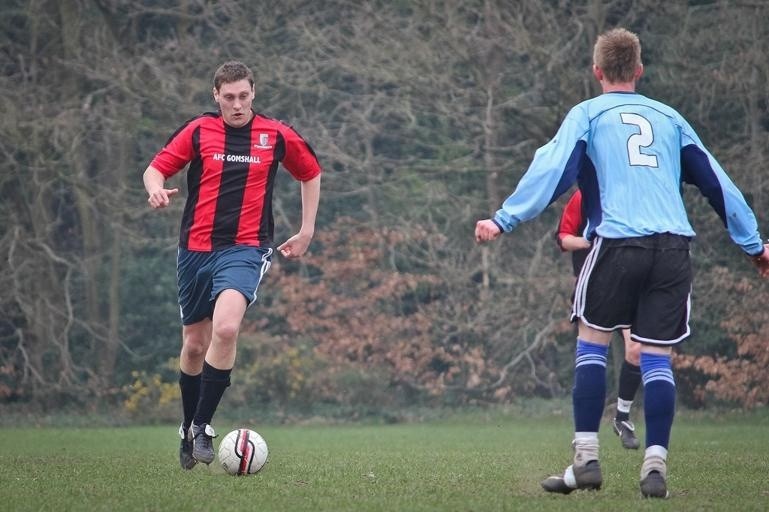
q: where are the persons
[140,58,323,470]
[473,24,769,498]
[555,188,646,452]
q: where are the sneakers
[179,422,219,470]
[640,459,666,498]
[541,459,602,494]
[612,417,640,450]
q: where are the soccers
[218,429,268,475]
